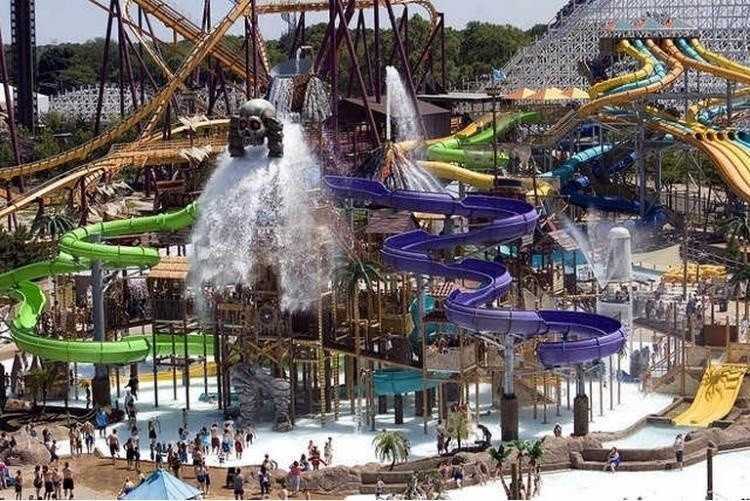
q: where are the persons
[475,441,486,448]
[469,459,489,478]
[444,437,451,453]
[0,288,333,500]
[436,420,446,433]
[381,428,391,462]
[603,446,620,472]
[622,285,629,300]
[360,289,401,304]
[478,425,492,443]
[436,433,444,454]
[386,460,464,500]
[401,440,410,464]
[524,440,531,447]
[645,283,710,327]
[672,433,684,471]
[375,475,385,500]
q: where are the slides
[386,112,555,204]
[518,39,750,146]
[373,295,457,395]
[1,199,200,366]
[721,129,749,156]
[646,114,749,202]
[670,362,746,428]
[322,176,627,367]
[555,139,685,240]
[712,129,749,170]
[685,101,750,187]
[425,110,555,173]
[687,100,750,147]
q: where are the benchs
[569,435,718,470]
[360,460,489,493]
